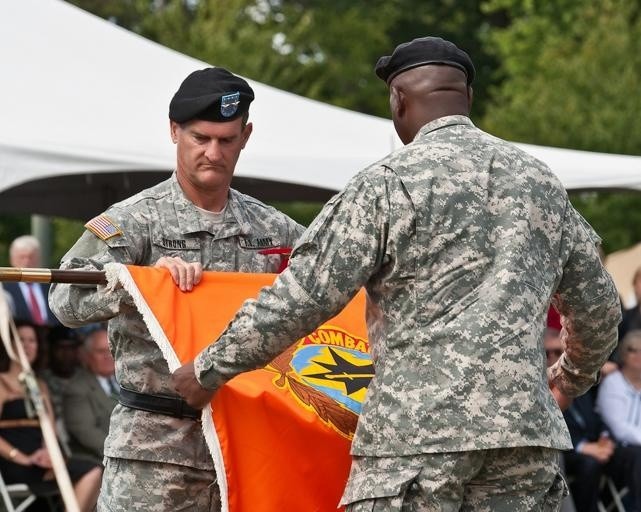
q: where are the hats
[375,36,476,88]
[169,67,255,123]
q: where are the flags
[104,262,376,512]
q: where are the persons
[45,68,308,511]
[542,246,641,512]
[172,35,626,512]
[0,236,120,510]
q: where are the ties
[25,281,44,326]
[108,377,121,409]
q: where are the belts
[119,385,201,420]
